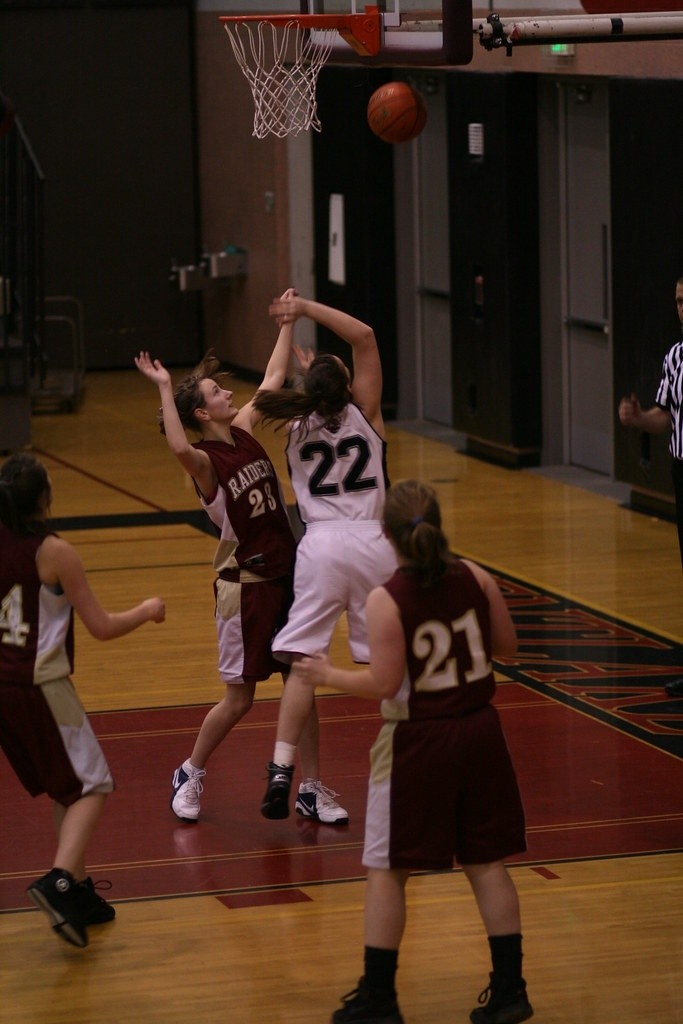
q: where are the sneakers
[332,975,404,1024]
[24,869,115,948]
[469,972,534,1024]
[295,781,348,825]
[261,761,295,820]
[169,758,206,822]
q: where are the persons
[258,294,406,820]
[133,344,350,826]
[287,477,534,1024]
[618,270,683,698]
[0,449,168,953]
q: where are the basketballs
[367,82,428,144]
